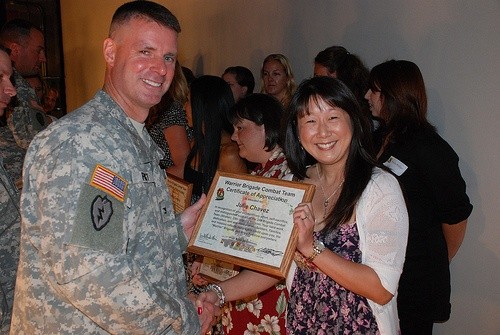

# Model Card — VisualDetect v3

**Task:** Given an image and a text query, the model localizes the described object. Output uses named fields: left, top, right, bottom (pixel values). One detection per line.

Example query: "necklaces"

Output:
left=315, top=163, right=344, bottom=219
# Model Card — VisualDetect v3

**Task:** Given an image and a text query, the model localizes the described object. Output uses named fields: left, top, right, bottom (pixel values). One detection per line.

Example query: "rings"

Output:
left=301, top=213, right=309, bottom=221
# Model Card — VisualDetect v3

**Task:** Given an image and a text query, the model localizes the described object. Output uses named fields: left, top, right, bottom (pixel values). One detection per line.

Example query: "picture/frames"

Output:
left=186, top=170, right=316, bottom=279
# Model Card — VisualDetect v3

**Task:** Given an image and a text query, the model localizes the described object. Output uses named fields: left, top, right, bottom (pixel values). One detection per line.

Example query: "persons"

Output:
left=143, top=45, right=472, bottom=335
left=10, top=0, right=222, bottom=335
left=0, top=19, right=65, bottom=335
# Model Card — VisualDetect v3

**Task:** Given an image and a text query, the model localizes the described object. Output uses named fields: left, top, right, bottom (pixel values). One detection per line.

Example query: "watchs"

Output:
left=306, top=240, right=326, bottom=262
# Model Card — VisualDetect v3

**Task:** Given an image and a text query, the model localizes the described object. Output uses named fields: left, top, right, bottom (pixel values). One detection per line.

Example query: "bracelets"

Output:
left=205, top=283, right=225, bottom=308
